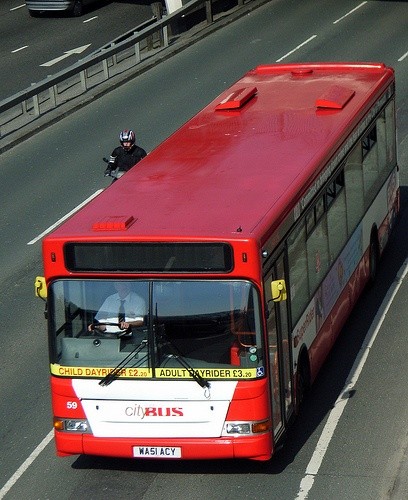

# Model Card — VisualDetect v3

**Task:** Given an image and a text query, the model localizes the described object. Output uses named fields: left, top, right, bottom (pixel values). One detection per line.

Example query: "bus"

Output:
left=33, top=61, right=401, bottom=461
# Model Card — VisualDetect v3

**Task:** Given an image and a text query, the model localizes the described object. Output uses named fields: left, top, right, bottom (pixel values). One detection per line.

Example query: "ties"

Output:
left=118, top=300, right=126, bottom=330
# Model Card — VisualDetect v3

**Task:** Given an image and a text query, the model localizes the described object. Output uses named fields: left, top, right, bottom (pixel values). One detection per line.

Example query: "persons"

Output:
left=88, top=279, right=146, bottom=335
left=104, top=130, right=147, bottom=184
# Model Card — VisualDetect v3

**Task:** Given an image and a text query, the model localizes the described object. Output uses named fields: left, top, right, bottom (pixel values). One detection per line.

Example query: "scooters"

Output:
left=103, top=157, right=133, bottom=184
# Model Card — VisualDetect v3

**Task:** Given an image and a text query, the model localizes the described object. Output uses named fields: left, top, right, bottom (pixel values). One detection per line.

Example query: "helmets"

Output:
left=119, top=129, right=135, bottom=151
